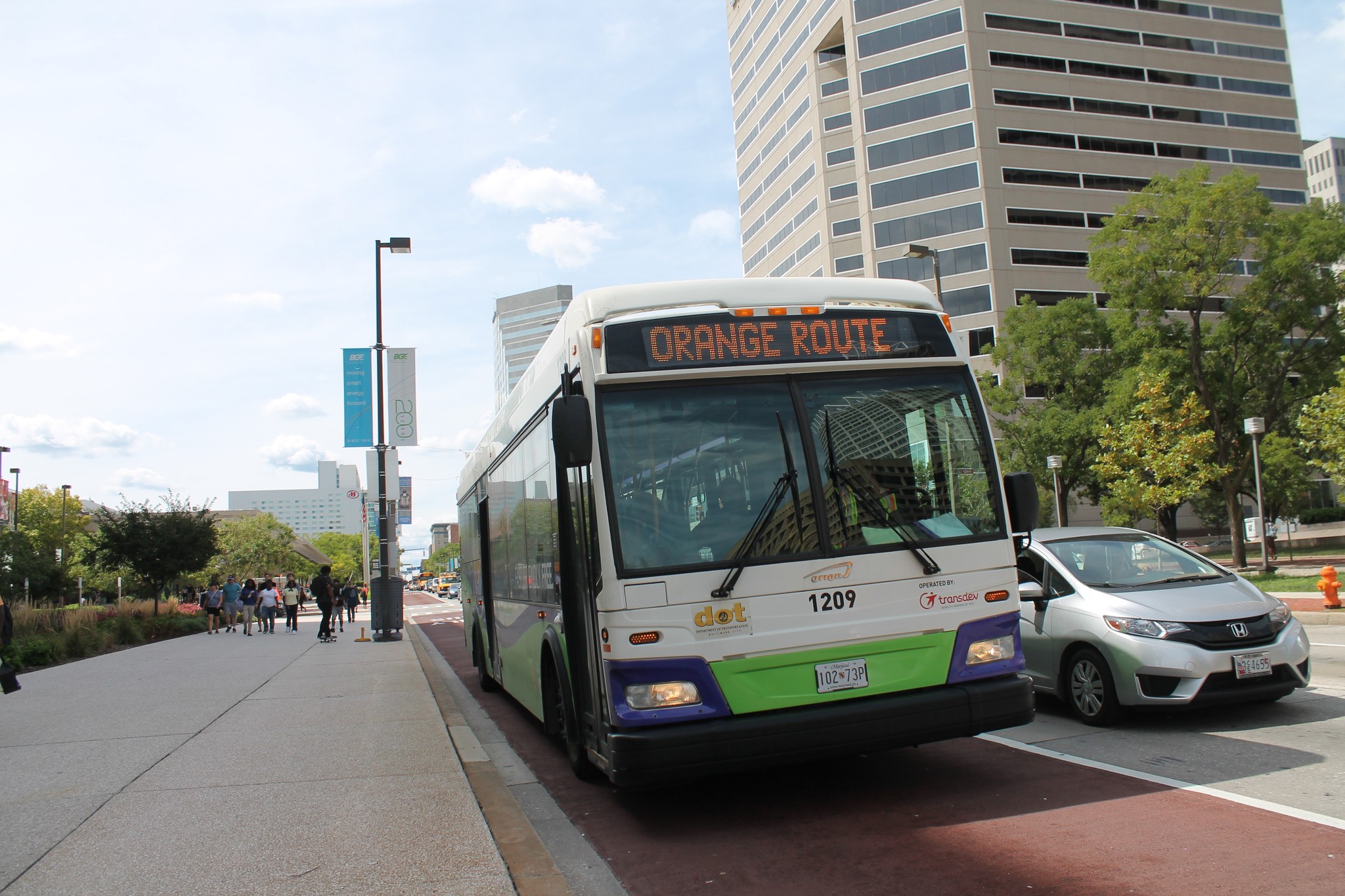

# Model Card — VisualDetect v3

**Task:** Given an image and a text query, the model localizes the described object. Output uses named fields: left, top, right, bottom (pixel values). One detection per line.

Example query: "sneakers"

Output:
left=326, top=635, right=337, bottom=639
left=317, top=635, right=326, bottom=640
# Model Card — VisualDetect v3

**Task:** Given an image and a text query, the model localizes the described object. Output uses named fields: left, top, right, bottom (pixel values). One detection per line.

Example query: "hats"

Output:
left=228, top=575, right=234, bottom=579
left=209, top=583, right=216, bottom=586
left=286, top=571, right=294, bottom=577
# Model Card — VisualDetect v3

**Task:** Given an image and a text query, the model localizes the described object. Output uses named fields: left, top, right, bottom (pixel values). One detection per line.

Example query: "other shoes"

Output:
left=271, top=630, right=274, bottom=633
left=244, top=627, right=247, bottom=635
left=226, top=626, right=231, bottom=633
left=292, top=630, right=296, bottom=634
left=248, top=633, right=252, bottom=636
left=269, top=630, right=271, bottom=632
left=352, top=616, right=355, bottom=623
left=233, top=628, right=236, bottom=632
left=263, top=629, right=269, bottom=633
left=330, top=628, right=335, bottom=632
left=286, top=626, right=290, bottom=632
left=215, top=631, right=219, bottom=634
left=208, top=631, right=212, bottom=634
left=340, top=628, right=344, bottom=632
left=258, top=630, right=262, bottom=632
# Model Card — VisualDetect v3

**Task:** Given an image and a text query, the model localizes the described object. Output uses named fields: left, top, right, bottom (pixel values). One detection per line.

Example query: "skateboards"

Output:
left=320, top=636, right=337, bottom=643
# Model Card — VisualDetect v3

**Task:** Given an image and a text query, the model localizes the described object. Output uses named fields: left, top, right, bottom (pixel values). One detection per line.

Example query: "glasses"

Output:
left=840, top=467, right=854, bottom=472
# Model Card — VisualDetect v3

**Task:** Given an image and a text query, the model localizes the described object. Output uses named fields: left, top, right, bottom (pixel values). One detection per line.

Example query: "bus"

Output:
left=456, top=276, right=1043, bottom=789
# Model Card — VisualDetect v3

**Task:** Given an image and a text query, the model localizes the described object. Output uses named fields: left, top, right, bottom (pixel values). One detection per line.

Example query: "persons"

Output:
left=826, top=468, right=902, bottom=551
left=1098, top=545, right=1149, bottom=582
left=1264, top=517, right=1278, bottom=560
left=692, top=478, right=759, bottom=562
left=202, top=565, right=368, bottom=639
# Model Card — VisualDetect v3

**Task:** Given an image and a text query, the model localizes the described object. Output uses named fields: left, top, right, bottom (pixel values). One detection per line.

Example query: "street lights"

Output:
left=1047, top=455, right=1065, bottom=528
left=10, top=468, right=21, bottom=561
left=1243, top=416, right=1279, bottom=573
left=902, top=244, right=946, bottom=311
left=60, top=485, right=72, bottom=608
left=0, top=446, right=11, bottom=479
left=374, top=237, right=412, bottom=644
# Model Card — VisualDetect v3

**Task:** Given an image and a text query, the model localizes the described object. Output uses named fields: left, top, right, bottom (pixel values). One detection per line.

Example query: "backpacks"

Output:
left=310, top=578, right=323, bottom=595
left=335, top=594, right=344, bottom=606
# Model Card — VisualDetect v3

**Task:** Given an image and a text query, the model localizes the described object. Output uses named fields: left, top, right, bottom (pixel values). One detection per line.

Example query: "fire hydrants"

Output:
left=1315, top=563, right=1343, bottom=609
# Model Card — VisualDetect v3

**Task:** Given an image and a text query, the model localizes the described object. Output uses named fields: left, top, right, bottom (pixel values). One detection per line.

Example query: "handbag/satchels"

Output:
left=204, top=608, right=206, bottom=611
left=360, top=590, right=365, bottom=598
left=235, top=598, right=244, bottom=612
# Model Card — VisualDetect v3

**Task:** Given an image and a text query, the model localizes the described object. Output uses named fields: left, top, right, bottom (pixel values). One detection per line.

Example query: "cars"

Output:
left=403, top=572, right=463, bottom=604
left=1013, top=525, right=1312, bottom=726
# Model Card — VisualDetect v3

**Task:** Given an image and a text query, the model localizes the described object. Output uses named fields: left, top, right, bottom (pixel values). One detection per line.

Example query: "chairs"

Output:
left=1017, top=556, right=1043, bottom=589
left=1081, top=547, right=1110, bottom=581
